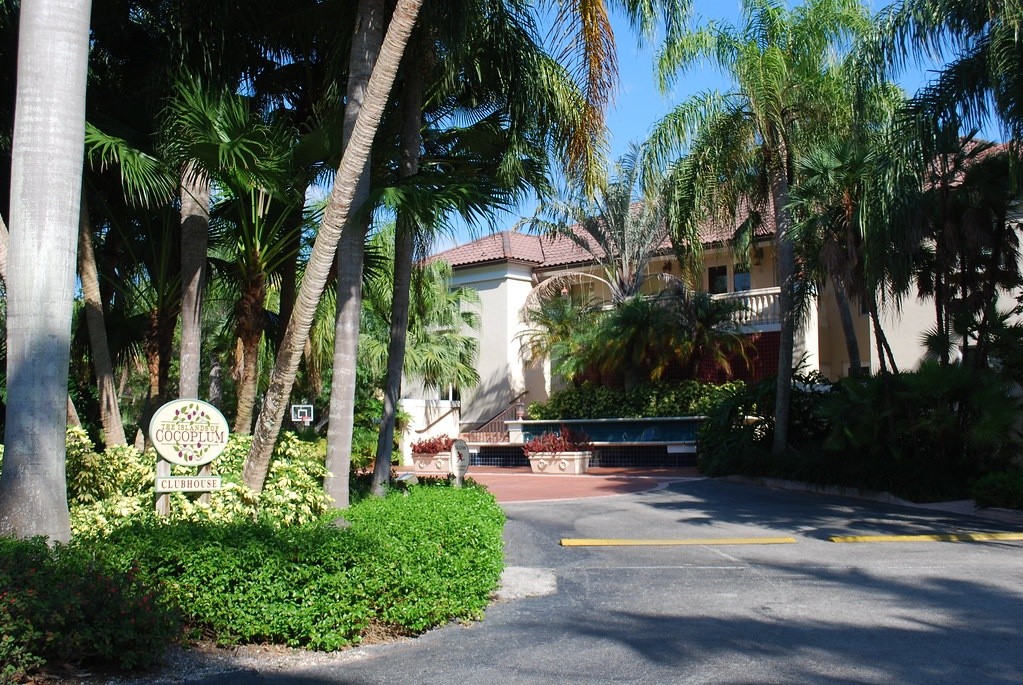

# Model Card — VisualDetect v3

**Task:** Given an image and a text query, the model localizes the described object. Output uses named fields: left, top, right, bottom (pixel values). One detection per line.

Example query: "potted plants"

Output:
left=409, top=433, right=457, bottom=472
left=521, top=421, right=594, bottom=475
